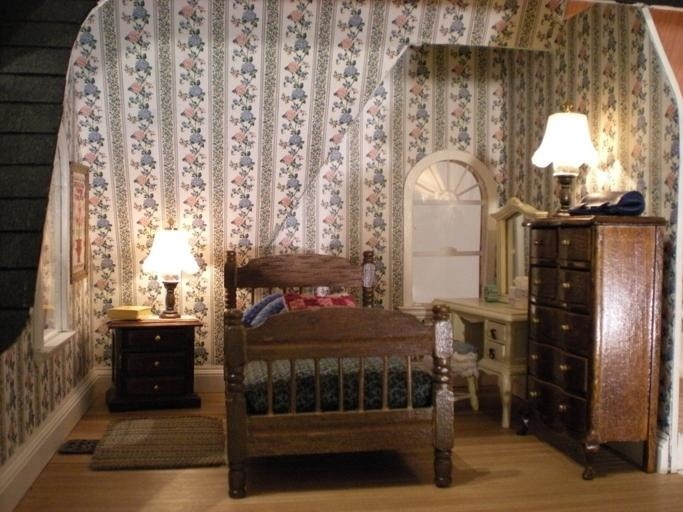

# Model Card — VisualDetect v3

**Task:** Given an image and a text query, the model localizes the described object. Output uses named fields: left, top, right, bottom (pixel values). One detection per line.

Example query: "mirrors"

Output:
left=492, top=198, right=547, bottom=298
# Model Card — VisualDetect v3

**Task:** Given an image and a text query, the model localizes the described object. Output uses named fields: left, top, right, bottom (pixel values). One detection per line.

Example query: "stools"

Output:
left=452, top=340, right=479, bottom=411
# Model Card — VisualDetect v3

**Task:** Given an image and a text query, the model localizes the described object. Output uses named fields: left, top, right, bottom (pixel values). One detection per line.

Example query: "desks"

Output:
left=434, top=298, right=528, bottom=429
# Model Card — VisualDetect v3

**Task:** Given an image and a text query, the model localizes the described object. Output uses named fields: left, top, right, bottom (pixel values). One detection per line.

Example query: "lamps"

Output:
left=142, top=230, right=200, bottom=319
left=532, top=103, right=603, bottom=217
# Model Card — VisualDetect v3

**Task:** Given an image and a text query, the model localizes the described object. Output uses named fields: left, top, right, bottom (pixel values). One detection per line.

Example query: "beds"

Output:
left=224, top=250, right=456, bottom=497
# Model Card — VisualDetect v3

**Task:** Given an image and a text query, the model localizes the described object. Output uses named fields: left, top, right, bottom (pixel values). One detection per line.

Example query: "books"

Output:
left=106, top=303, right=154, bottom=322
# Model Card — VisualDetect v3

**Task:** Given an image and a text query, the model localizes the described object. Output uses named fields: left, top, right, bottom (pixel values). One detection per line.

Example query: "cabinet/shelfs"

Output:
left=516, top=215, right=667, bottom=480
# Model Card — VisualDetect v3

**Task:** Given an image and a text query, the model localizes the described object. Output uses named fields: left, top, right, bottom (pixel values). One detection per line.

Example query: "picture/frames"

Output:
left=70, top=161, right=89, bottom=284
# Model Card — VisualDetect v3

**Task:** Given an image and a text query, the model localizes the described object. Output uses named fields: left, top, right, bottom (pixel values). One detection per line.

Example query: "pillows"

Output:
left=281, top=293, right=358, bottom=309
left=242, top=294, right=283, bottom=327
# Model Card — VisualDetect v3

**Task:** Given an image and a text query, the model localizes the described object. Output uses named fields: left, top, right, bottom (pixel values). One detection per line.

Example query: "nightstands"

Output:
left=105, top=315, right=203, bottom=413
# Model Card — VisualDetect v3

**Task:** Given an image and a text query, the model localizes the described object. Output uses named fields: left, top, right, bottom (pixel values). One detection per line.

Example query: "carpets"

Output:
left=89, top=417, right=226, bottom=468
